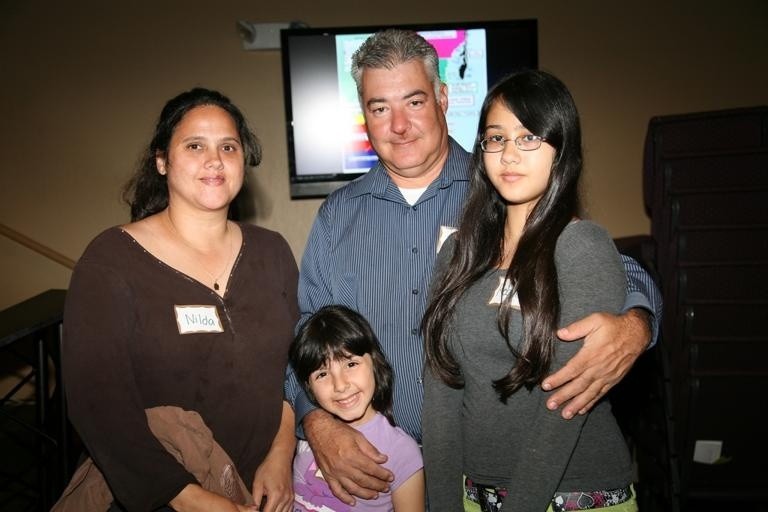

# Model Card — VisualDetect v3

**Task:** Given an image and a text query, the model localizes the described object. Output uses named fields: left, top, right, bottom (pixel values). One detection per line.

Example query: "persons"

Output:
left=422, top=70, right=638, bottom=509
left=284, top=30, right=663, bottom=512
left=54, top=88, right=299, bottom=512
left=290, top=306, right=425, bottom=512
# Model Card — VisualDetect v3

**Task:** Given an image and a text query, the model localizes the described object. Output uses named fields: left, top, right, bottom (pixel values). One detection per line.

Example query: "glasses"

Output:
left=480, top=135, right=544, bottom=152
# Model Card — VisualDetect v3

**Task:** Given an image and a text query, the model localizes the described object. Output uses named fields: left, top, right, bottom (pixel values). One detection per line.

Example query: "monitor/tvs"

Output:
left=277, top=16, right=539, bottom=200
